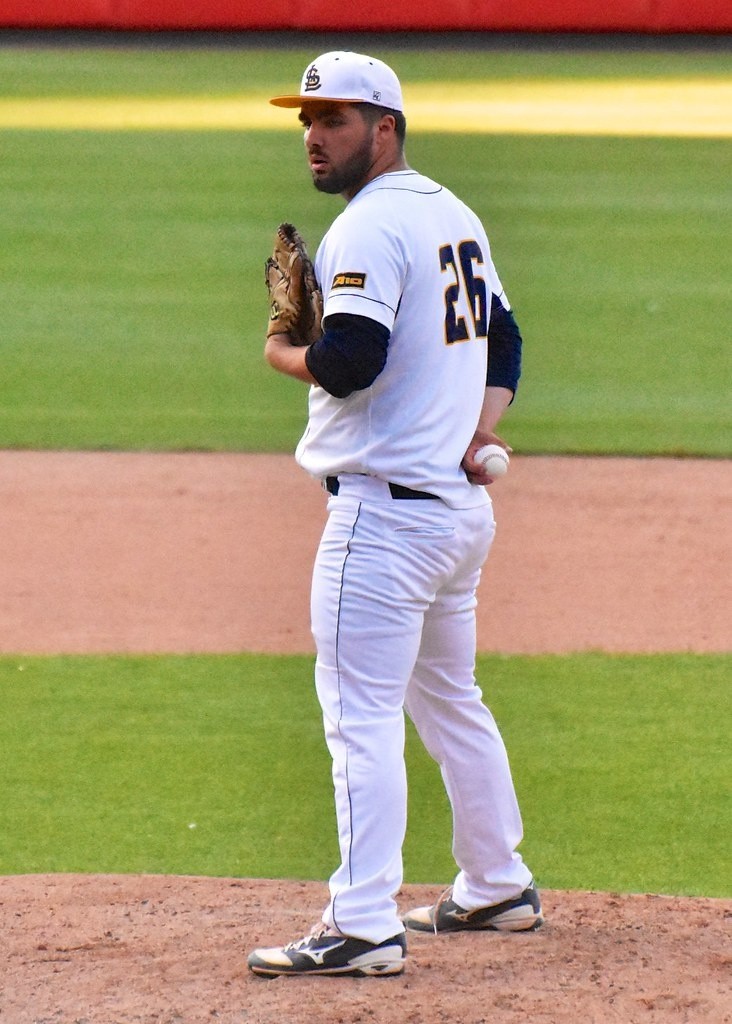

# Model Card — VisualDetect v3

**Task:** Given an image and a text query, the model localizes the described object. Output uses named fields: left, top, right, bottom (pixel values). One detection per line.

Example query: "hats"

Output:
left=268, top=51, right=403, bottom=112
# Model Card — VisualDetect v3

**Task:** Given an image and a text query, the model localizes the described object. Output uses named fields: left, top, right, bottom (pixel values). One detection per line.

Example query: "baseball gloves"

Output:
left=264, top=221, right=325, bottom=347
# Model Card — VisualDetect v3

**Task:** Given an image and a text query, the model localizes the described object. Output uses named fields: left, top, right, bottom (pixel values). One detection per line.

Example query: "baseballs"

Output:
left=473, top=443, right=509, bottom=479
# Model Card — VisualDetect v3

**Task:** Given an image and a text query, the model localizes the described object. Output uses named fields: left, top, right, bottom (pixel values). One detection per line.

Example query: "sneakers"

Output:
left=402, top=878, right=544, bottom=932
left=246, top=920, right=407, bottom=977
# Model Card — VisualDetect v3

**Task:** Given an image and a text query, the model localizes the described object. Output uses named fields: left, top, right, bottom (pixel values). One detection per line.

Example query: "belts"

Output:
left=321, top=476, right=441, bottom=500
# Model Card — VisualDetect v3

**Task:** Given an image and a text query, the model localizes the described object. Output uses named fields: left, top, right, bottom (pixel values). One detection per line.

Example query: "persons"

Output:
left=246, top=51, right=543, bottom=979
